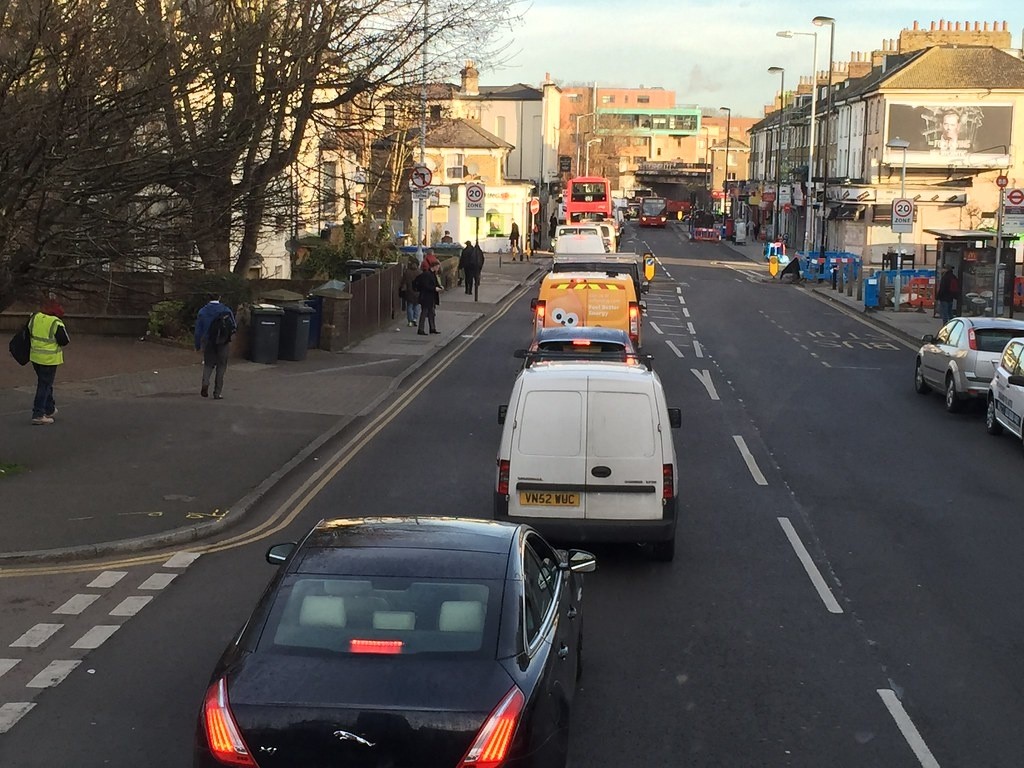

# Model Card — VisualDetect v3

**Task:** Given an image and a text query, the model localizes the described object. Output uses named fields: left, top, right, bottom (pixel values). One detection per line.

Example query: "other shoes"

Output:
left=430, top=331, right=441, bottom=334
left=32, top=415, right=55, bottom=424
left=201, top=383, right=208, bottom=397
left=465, top=291, right=468, bottom=294
left=47, top=408, right=59, bottom=418
left=214, top=395, right=223, bottom=399
left=417, top=330, right=428, bottom=335
left=469, top=292, right=472, bottom=295
left=408, top=321, right=412, bottom=327
left=412, top=318, right=417, bottom=326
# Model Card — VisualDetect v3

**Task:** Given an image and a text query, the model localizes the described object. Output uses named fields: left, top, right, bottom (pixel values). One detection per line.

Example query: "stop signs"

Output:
left=531, top=200, right=539, bottom=215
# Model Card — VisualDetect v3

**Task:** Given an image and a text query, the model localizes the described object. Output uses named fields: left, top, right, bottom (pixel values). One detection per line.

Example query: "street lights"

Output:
left=577, top=111, right=601, bottom=177
left=701, top=107, right=730, bottom=233
left=886, top=137, right=910, bottom=312
left=768, top=66, right=785, bottom=242
left=776, top=16, right=835, bottom=282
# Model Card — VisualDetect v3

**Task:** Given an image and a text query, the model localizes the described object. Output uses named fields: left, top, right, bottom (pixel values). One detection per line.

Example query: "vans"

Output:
left=493, top=350, right=682, bottom=561
left=530, top=269, right=647, bottom=350
left=613, top=200, right=624, bottom=232
left=555, top=218, right=619, bottom=253
left=552, top=234, right=608, bottom=254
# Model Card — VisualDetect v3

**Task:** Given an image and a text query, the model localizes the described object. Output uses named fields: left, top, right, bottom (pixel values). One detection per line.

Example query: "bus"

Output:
left=566, top=177, right=612, bottom=225
left=639, top=196, right=667, bottom=228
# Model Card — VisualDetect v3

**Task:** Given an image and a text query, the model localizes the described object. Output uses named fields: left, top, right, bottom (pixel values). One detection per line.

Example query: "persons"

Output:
left=550, top=213, right=557, bottom=237
left=938, top=264, right=957, bottom=325
left=509, top=218, right=520, bottom=253
left=927, top=110, right=969, bottom=151
left=417, top=261, right=444, bottom=336
left=754, top=223, right=760, bottom=240
left=27, top=299, right=70, bottom=425
left=458, top=241, right=484, bottom=295
left=420, top=249, right=438, bottom=272
left=194, top=288, right=237, bottom=398
left=400, top=258, right=422, bottom=326
left=442, top=231, right=453, bottom=244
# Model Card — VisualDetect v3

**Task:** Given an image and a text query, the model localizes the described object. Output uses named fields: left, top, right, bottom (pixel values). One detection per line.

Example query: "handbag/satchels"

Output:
left=399, top=282, right=407, bottom=297
left=457, top=269, right=462, bottom=285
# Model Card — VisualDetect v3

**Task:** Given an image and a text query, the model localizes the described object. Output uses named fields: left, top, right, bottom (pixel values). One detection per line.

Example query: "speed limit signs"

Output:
left=465, top=183, right=485, bottom=217
left=892, top=198, right=913, bottom=233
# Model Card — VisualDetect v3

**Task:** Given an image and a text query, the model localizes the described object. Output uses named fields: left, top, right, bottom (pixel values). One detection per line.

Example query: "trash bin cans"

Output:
left=282, top=305, right=315, bottom=360
left=725, top=219, right=733, bottom=240
left=434, top=242, right=464, bottom=285
left=250, top=304, right=285, bottom=363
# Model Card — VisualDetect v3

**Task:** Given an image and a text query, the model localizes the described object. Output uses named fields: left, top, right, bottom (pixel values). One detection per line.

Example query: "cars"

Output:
left=913, top=316, right=1024, bottom=444
left=194, top=513, right=597, bottom=768
left=514, top=328, right=652, bottom=372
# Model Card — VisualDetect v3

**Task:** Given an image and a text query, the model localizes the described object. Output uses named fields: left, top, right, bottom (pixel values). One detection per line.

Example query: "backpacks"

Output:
left=9, top=312, right=38, bottom=366
left=210, top=303, right=235, bottom=353
left=412, top=273, right=424, bottom=292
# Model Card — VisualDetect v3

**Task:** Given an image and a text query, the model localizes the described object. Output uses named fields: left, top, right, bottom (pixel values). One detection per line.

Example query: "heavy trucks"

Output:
left=624, top=186, right=692, bottom=221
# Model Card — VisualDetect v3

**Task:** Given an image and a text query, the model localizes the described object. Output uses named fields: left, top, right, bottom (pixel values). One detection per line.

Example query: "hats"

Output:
left=464, top=241, right=472, bottom=245
left=944, top=263, right=953, bottom=271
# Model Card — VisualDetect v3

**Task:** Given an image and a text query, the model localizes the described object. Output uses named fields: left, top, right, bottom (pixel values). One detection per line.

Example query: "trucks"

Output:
left=549, top=252, right=649, bottom=305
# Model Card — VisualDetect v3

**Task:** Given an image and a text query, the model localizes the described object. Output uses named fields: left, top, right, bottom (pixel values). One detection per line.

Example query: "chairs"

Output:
left=323, top=581, right=392, bottom=624
